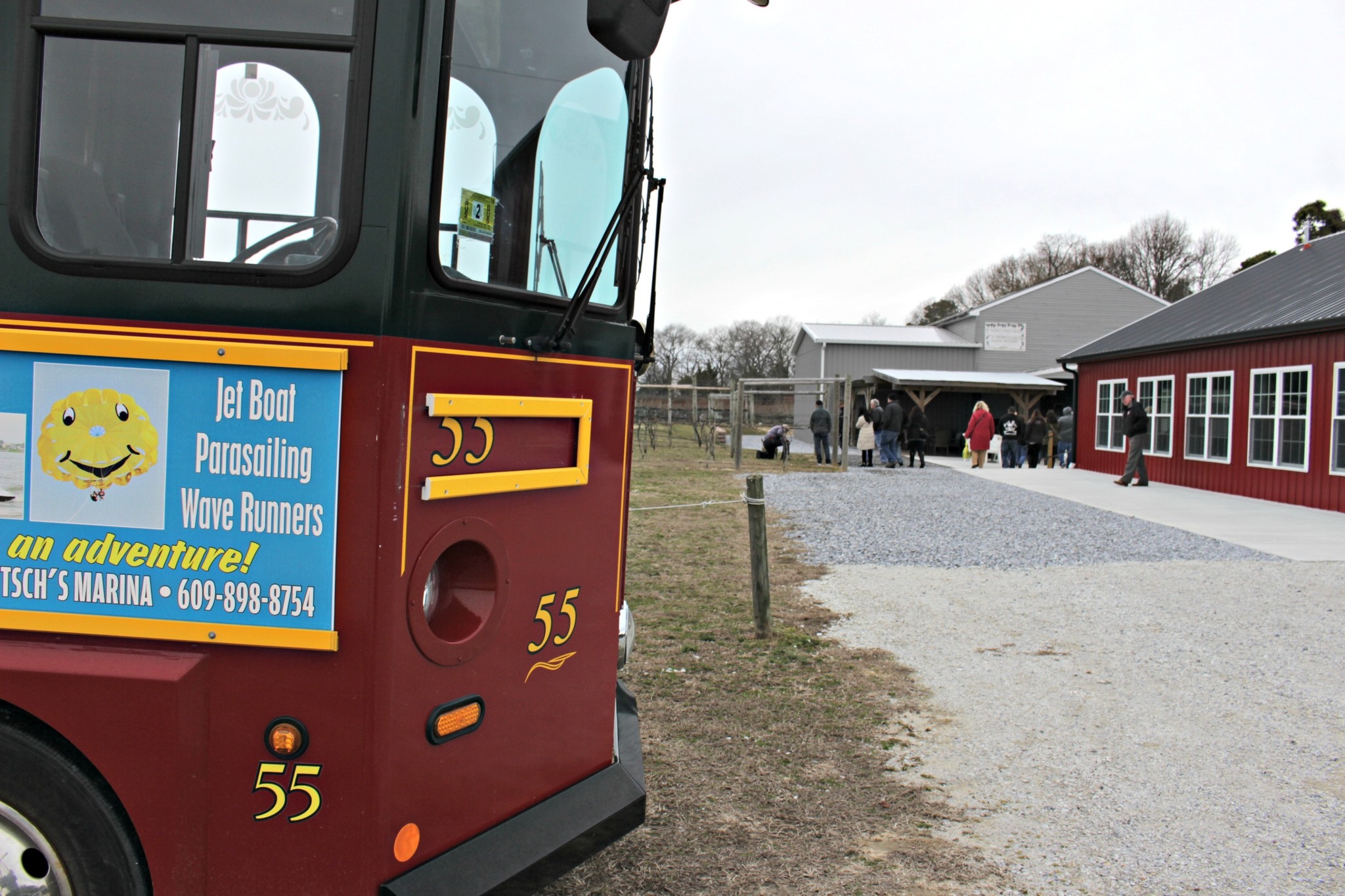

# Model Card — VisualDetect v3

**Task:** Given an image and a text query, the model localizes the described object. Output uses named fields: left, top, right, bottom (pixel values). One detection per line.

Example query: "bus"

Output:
left=0, top=0, right=679, bottom=896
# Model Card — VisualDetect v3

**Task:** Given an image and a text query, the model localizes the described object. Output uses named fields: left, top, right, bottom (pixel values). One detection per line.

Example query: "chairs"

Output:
left=932, top=432, right=961, bottom=455
left=985, top=435, right=1002, bottom=464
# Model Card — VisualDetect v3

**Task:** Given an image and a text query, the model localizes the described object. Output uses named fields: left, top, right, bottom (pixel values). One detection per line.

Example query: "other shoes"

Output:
left=919, top=463, right=924, bottom=468
left=886, top=461, right=898, bottom=468
left=1061, top=463, right=1066, bottom=468
left=1114, top=480, right=1128, bottom=487
left=825, top=463, right=831, bottom=465
left=1133, top=483, right=1147, bottom=486
left=858, top=463, right=873, bottom=467
left=817, top=463, right=821, bottom=465
left=756, top=450, right=761, bottom=458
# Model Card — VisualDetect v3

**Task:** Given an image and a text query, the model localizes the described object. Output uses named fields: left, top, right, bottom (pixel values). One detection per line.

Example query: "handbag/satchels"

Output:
left=920, top=429, right=928, bottom=438
left=963, top=439, right=971, bottom=460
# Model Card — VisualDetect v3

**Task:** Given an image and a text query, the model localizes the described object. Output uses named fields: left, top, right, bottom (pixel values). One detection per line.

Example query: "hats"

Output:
left=1118, top=391, right=1132, bottom=399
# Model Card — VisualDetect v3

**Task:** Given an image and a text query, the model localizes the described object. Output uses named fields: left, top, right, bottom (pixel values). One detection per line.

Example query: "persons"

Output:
left=753, top=394, right=1075, bottom=469
left=1113, top=391, right=1151, bottom=486
